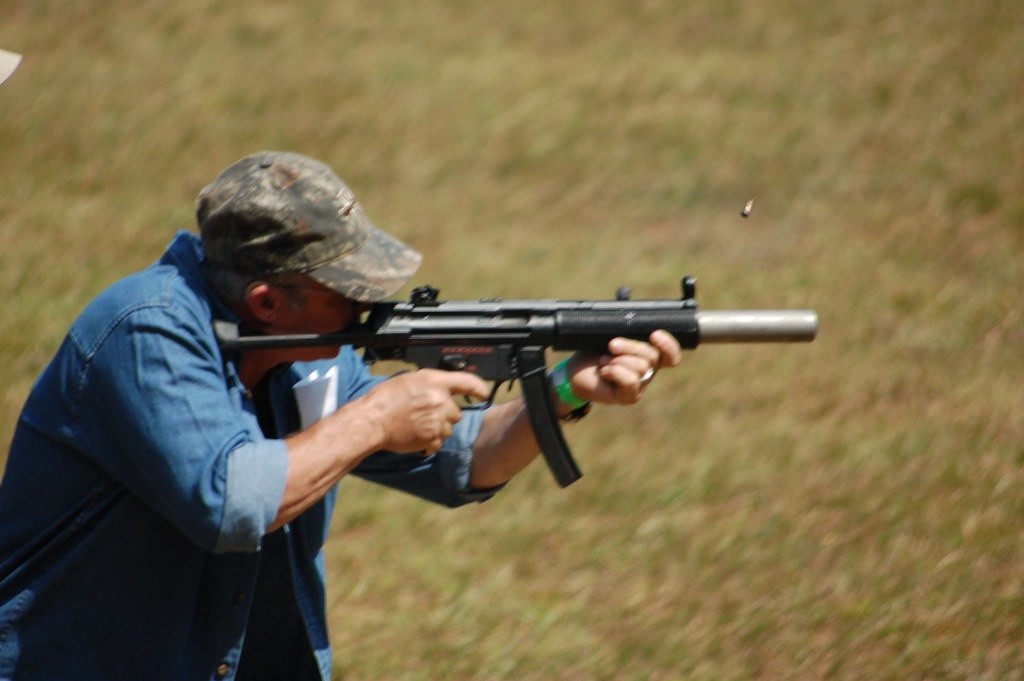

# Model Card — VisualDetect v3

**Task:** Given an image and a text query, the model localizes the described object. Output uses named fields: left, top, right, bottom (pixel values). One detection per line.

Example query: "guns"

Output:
left=212, top=275, right=820, bottom=489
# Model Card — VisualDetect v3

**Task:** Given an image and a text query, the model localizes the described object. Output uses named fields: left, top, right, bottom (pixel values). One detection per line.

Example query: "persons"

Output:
left=0, top=151, right=685, bottom=681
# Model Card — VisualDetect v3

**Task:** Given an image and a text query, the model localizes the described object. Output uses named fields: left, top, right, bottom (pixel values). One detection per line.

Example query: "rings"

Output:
left=641, top=368, right=655, bottom=385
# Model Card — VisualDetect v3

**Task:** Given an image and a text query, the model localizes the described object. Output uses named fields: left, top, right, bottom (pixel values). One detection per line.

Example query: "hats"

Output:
left=193, top=151, right=425, bottom=305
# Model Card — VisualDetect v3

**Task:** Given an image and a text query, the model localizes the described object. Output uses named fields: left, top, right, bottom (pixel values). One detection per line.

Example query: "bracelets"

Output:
left=552, top=357, right=588, bottom=411
